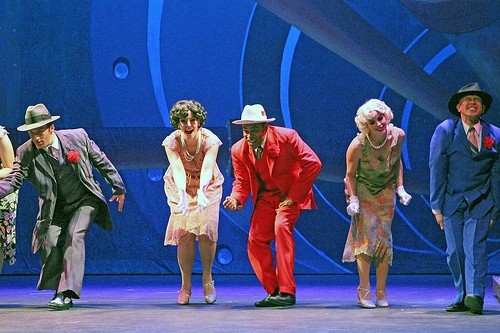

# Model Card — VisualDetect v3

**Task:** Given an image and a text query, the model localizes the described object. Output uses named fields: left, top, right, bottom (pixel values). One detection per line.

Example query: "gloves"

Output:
left=347, top=196, right=360, bottom=215
left=396, top=186, right=412, bottom=206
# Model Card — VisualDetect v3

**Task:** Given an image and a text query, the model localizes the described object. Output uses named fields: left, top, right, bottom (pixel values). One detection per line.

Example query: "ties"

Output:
left=468, top=128, right=478, bottom=149
left=48, top=147, right=59, bottom=160
left=256, top=146, right=261, bottom=160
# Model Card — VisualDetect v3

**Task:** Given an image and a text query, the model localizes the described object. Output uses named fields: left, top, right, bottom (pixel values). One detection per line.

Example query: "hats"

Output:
left=448, top=82, right=493, bottom=117
left=16, top=103, right=61, bottom=132
left=231, top=104, right=275, bottom=124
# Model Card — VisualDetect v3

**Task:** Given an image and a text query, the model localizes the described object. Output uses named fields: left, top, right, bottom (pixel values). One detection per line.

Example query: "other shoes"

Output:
left=202, top=281, right=217, bottom=304
left=177, top=289, right=191, bottom=305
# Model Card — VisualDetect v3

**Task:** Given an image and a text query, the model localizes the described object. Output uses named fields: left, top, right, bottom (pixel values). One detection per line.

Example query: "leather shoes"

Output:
left=446, top=303, right=467, bottom=312
left=269, top=292, right=295, bottom=307
left=255, top=294, right=276, bottom=307
left=47, top=292, right=73, bottom=310
left=465, top=294, right=484, bottom=315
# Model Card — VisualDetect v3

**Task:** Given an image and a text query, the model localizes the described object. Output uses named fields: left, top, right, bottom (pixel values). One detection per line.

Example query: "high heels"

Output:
left=377, top=289, right=389, bottom=306
left=356, top=286, right=376, bottom=308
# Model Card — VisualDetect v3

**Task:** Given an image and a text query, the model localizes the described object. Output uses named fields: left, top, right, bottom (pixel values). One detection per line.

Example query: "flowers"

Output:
left=268, top=144, right=280, bottom=158
left=67, top=150, right=79, bottom=162
left=483, top=136, right=494, bottom=148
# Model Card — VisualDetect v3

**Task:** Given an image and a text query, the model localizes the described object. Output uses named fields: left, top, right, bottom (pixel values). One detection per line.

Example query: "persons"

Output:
left=162, top=99, right=225, bottom=305
left=429, top=82, right=500, bottom=315
left=342, top=98, right=413, bottom=308
left=0, top=104, right=126, bottom=311
left=223, top=103, right=322, bottom=307
left=0, top=125, right=19, bottom=275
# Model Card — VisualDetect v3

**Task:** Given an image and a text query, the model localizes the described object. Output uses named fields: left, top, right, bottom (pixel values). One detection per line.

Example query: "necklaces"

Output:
left=366, top=127, right=388, bottom=149
left=179, top=129, right=201, bottom=161
